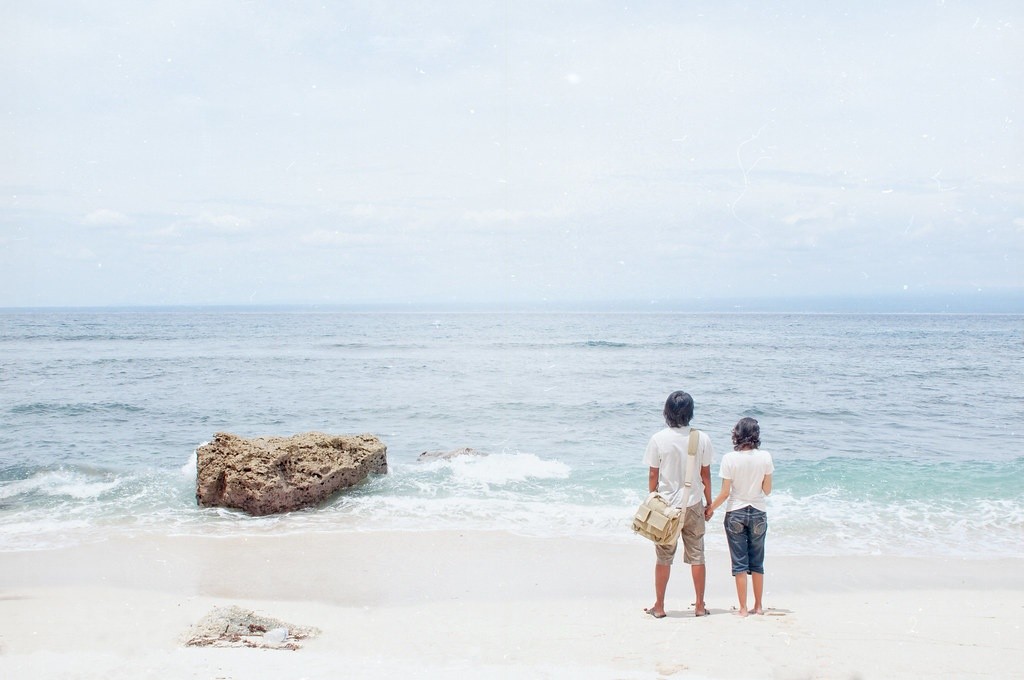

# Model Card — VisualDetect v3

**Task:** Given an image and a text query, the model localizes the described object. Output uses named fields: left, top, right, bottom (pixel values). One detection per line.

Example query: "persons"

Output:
left=706, top=417, right=775, bottom=617
left=646, top=391, right=714, bottom=618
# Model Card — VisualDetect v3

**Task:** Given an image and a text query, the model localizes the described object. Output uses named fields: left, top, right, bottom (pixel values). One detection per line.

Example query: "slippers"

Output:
left=695, top=609, right=710, bottom=617
left=646, top=608, right=666, bottom=619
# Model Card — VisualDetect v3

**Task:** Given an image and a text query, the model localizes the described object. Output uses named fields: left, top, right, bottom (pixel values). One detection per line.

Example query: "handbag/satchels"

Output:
left=631, top=490, right=685, bottom=545
